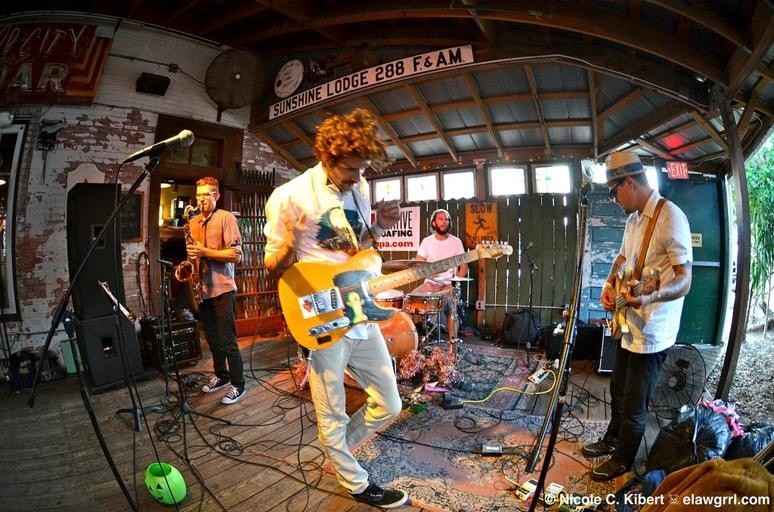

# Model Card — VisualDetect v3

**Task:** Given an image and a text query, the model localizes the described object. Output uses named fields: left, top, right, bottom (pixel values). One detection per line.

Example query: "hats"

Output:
left=606, top=152, right=645, bottom=180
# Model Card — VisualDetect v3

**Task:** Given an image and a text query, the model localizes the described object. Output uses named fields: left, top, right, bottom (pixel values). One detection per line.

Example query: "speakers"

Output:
left=66, top=183, right=145, bottom=394
left=136, top=72, right=170, bottom=96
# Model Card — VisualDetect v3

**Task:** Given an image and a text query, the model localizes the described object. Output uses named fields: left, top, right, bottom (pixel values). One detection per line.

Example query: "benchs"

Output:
left=573, top=322, right=604, bottom=360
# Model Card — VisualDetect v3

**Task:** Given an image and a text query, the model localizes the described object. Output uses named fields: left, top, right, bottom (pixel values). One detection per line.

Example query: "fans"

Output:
left=206, top=46, right=269, bottom=122
left=649, top=340, right=706, bottom=431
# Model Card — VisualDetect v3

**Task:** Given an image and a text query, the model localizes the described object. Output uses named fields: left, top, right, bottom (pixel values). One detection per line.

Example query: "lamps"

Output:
left=0, top=107, right=16, bottom=128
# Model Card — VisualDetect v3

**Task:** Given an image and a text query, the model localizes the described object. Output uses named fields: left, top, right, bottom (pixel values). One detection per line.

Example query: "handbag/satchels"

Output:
left=544, top=317, right=585, bottom=358
left=503, top=308, right=540, bottom=346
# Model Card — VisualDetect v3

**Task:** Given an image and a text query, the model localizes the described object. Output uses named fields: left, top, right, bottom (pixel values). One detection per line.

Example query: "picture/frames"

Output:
left=120, top=191, right=144, bottom=243
left=465, top=202, right=500, bottom=251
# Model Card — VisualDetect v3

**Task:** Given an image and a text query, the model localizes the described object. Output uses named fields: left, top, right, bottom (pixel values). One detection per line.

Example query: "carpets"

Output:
left=294, top=341, right=547, bottom=413
left=318, top=400, right=650, bottom=512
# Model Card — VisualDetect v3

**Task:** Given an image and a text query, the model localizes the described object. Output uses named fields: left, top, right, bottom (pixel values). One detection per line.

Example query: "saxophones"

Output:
left=174, top=204, right=205, bottom=314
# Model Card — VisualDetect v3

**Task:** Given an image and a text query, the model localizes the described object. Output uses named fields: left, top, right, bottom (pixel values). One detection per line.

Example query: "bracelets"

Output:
left=371, top=222, right=385, bottom=234
left=601, top=282, right=613, bottom=290
left=274, top=252, right=295, bottom=270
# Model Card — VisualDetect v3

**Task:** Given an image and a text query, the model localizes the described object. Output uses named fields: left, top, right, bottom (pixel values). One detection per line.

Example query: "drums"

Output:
left=405, top=292, right=447, bottom=315
left=344, top=307, right=419, bottom=381
left=375, top=289, right=404, bottom=309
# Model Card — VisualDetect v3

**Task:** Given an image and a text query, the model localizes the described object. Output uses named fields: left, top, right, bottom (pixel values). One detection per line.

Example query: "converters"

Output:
left=481, top=443, right=502, bottom=456
left=443, top=393, right=452, bottom=402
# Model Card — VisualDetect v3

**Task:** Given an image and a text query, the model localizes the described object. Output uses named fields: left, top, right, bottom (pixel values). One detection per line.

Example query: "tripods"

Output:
left=157, top=286, right=231, bottom=459
left=116, top=318, right=161, bottom=432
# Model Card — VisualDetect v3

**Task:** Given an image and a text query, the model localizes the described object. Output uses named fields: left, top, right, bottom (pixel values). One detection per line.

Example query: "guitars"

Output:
left=611, top=268, right=660, bottom=341
left=277, top=240, right=513, bottom=349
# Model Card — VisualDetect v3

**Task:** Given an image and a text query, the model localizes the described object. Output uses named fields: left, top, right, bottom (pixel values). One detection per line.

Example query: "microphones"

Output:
left=524, top=241, right=533, bottom=252
left=123, top=129, right=195, bottom=164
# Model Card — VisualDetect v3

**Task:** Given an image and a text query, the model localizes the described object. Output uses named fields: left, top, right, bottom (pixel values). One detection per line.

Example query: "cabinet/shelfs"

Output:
left=224, top=184, right=283, bottom=338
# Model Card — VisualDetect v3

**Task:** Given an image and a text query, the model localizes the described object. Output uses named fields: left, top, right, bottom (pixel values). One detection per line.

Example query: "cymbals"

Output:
left=381, top=257, right=431, bottom=272
left=449, top=277, right=474, bottom=281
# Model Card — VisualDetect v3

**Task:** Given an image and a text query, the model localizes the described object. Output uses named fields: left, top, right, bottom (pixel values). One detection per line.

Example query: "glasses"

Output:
left=609, top=176, right=634, bottom=199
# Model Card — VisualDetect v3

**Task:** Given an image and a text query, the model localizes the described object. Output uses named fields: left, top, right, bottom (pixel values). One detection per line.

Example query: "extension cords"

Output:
left=528, top=367, right=551, bottom=385
left=442, top=398, right=463, bottom=409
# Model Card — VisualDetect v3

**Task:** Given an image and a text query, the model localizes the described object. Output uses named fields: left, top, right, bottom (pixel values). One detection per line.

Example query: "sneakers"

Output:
left=590, top=459, right=631, bottom=479
left=350, top=482, right=407, bottom=508
left=221, top=386, right=245, bottom=404
left=202, top=376, right=230, bottom=393
left=581, top=439, right=618, bottom=455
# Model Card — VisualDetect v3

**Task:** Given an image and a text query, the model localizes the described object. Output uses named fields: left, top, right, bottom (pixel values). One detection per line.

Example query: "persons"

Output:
left=263, top=109, right=407, bottom=510
left=186, top=177, right=246, bottom=405
left=582, top=151, right=694, bottom=481
left=416, top=209, right=470, bottom=360
